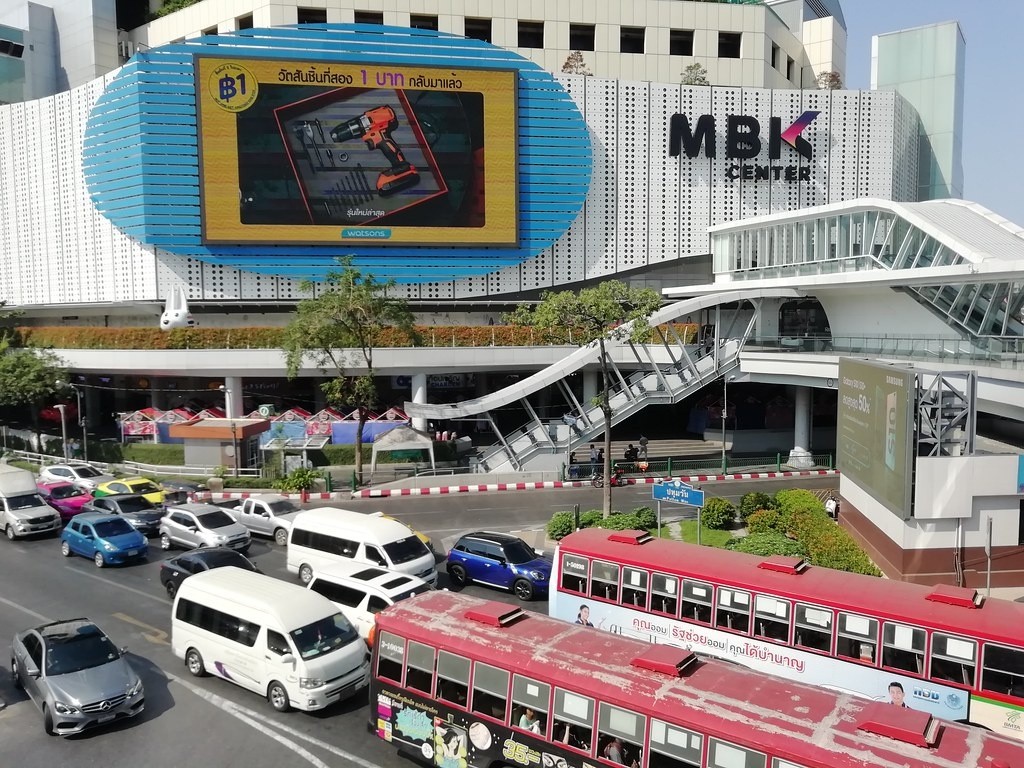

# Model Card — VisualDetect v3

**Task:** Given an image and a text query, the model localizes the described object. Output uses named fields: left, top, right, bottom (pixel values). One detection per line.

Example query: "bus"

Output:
left=365, top=587, right=1024, bottom=768
left=548, top=527, right=1024, bottom=746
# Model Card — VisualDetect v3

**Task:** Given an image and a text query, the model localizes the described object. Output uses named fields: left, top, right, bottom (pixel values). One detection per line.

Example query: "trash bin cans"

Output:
left=570, top=466, right=580, bottom=477
left=780, top=338, right=803, bottom=352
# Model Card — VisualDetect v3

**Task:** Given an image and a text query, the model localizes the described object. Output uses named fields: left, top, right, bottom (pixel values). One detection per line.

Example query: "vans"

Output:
left=286, top=506, right=439, bottom=588
left=0, top=462, right=63, bottom=541
left=169, top=563, right=372, bottom=713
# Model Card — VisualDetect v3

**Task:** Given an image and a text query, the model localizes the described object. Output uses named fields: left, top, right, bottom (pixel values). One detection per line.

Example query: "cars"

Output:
left=36, top=479, right=96, bottom=522
left=80, top=493, right=166, bottom=539
left=59, top=512, right=149, bottom=568
left=94, top=476, right=177, bottom=506
left=157, top=502, right=253, bottom=554
left=159, top=477, right=206, bottom=502
left=159, top=546, right=266, bottom=599
left=447, top=530, right=554, bottom=601
left=366, top=511, right=434, bottom=553
left=9, top=616, right=146, bottom=736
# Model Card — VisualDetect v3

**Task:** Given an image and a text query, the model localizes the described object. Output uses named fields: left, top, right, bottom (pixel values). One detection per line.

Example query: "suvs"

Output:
left=36, top=461, right=118, bottom=494
left=305, top=559, right=438, bottom=654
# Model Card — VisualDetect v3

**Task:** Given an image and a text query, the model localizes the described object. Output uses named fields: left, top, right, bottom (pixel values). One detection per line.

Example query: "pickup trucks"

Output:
left=190, top=493, right=309, bottom=547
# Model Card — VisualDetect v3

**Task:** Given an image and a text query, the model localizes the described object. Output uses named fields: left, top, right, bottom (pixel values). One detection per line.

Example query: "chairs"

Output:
left=579, top=578, right=971, bottom=687
left=407, top=667, right=616, bottom=759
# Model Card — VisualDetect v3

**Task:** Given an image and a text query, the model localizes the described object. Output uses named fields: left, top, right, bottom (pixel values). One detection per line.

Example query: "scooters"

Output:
left=592, top=465, right=625, bottom=489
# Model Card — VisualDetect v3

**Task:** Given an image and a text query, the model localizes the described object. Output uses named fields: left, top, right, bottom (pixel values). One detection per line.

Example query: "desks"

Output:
left=395, top=467, right=415, bottom=480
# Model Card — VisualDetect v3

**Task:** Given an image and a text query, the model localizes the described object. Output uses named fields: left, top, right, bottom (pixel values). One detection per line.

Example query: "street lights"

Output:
left=53, top=403, right=68, bottom=463
left=54, top=379, right=88, bottom=462
left=220, top=385, right=233, bottom=426
left=721, top=375, right=736, bottom=458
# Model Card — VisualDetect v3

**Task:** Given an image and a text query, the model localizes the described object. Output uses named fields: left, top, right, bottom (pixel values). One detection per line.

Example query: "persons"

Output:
left=627, top=433, right=648, bottom=462
left=589, top=444, right=604, bottom=477
left=610, top=737, right=638, bottom=768
left=575, top=604, right=593, bottom=626
left=888, top=682, right=912, bottom=709
left=570, top=452, right=576, bottom=464
left=559, top=722, right=588, bottom=749
left=519, top=706, right=540, bottom=730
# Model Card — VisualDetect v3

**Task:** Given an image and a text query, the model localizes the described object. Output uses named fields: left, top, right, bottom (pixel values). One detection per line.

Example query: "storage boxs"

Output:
left=273, top=87, right=448, bottom=226
